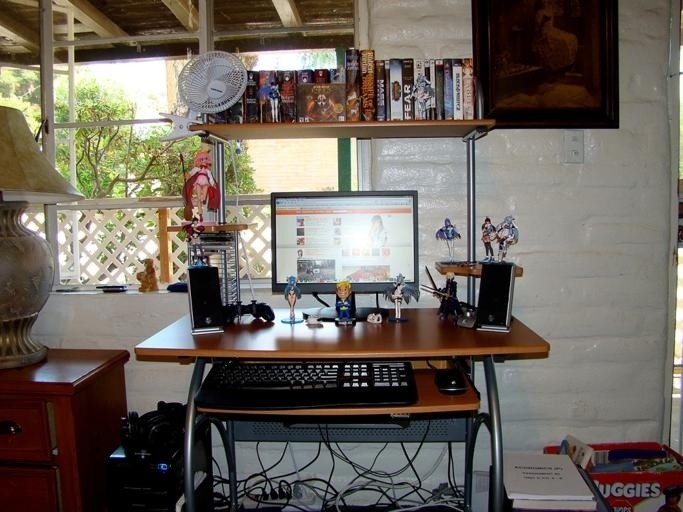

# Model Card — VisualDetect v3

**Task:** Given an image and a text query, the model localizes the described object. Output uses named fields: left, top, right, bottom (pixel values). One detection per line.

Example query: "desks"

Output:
left=133, top=307, right=551, bottom=512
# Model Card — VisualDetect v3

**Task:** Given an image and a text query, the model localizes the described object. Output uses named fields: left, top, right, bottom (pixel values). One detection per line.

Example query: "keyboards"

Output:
left=196, top=360, right=419, bottom=407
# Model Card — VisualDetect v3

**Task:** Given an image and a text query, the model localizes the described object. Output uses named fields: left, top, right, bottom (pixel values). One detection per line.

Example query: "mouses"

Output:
left=434, top=368, right=467, bottom=395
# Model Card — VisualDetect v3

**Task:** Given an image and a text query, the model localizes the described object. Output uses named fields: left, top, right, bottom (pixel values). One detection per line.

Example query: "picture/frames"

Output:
left=471, top=0, right=619, bottom=129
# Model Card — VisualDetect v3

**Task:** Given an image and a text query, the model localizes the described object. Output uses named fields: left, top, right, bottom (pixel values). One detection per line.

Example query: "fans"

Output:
left=160, top=51, right=248, bottom=141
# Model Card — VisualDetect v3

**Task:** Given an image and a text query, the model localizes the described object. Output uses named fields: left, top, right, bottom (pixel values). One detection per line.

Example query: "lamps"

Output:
left=2, top=105, right=85, bottom=372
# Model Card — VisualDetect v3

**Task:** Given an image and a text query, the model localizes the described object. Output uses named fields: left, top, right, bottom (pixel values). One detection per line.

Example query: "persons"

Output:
left=185, top=217, right=206, bottom=264
left=336, top=281, right=352, bottom=319
left=186, top=151, right=216, bottom=222
left=657, top=494, right=681, bottom=512
left=481, top=218, right=497, bottom=262
left=284, top=274, right=302, bottom=319
left=496, top=215, right=520, bottom=263
left=438, top=271, right=463, bottom=320
left=391, top=273, right=405, bottom=318
left=436, top=218, right=461, bottom=262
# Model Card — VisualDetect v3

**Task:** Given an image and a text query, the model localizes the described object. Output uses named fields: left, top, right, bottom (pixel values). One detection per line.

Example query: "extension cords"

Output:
left=243, top=491, right=315, bottom=509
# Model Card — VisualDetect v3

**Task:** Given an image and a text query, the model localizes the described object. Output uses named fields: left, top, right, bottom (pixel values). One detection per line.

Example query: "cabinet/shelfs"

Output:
left=1, top=348, right=129, bottom=510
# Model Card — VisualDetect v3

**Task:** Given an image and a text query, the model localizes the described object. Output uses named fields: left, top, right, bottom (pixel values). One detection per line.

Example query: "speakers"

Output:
left=187, top=266, right=224, bottom=336
left=476, top=262, right=515, bottom=333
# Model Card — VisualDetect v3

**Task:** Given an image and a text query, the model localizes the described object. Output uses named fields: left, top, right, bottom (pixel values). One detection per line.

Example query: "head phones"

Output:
left=121, top=401, right=185, bottom=453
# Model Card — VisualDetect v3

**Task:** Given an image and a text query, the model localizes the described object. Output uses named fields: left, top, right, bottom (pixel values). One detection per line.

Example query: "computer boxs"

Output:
left=105, top=422, right=215, bottom=512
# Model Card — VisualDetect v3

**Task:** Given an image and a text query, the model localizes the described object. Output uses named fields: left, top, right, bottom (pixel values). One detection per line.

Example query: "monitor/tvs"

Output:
left=271, top=189, right=419, bottom=321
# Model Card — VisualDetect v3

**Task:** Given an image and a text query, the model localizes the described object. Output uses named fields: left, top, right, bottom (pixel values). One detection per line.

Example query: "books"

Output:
left=503, top=433, right=597, bottom=512
left=215, top=48, right=476, bottom=124
